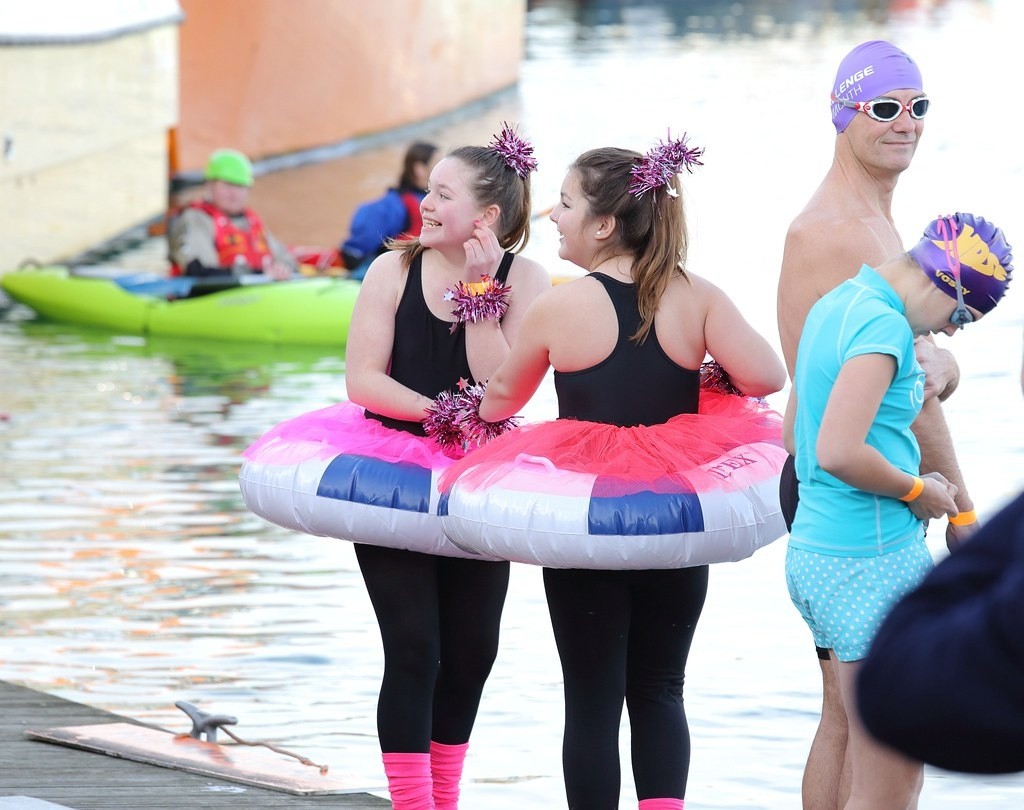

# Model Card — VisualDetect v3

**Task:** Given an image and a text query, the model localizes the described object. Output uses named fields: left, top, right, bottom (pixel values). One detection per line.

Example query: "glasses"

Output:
left=936, top=214, right=976, bottom=331
left=829, top=87, right=932, bottom=123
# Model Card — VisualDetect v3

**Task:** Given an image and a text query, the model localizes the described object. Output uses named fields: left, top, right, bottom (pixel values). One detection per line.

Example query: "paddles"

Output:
left=115, top=268, right=278, bottom=299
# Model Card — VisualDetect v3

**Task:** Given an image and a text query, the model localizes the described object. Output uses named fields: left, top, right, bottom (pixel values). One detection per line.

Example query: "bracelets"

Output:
left=899, top=474, right=925, bottom=503
left=947, top=507, right=978, bottom=527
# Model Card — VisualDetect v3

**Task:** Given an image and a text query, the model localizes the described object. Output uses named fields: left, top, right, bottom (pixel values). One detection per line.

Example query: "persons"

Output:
left=461, top=146, right=788, bottom=809
left=777, top=39, right=982, bottom=809
left=168, top=146, right=303, bottom=283
left=784, top=210, right=1016, bottom=809
left=344, top=144, right=554, bottom=809
left=337, top=139, right=446, bottom=282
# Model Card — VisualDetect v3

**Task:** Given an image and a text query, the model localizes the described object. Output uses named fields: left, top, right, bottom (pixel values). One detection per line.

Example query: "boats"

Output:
left=1, top=266, right=363, bottom=350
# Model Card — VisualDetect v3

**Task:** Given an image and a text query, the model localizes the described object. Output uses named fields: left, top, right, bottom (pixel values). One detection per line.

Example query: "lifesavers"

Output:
left=236, top=435, right=504, bottom=564
left=436, top=443, right=794, bottom=573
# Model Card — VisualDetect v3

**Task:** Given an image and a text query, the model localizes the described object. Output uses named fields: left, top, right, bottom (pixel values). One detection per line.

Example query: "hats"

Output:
left=909, top=212, right=1014, bottom=316
left=206, top=146, right=251, bottom=189
left=831, top=38, right=922, bottom=137
